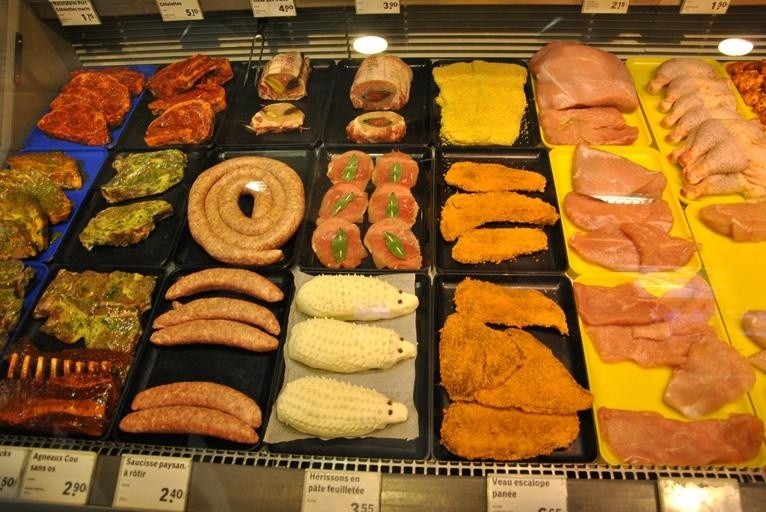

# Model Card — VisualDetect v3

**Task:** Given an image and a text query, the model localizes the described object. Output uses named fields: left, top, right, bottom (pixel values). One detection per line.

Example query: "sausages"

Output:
left=149, top=266, right=285, bottom=352
left=118, top=380, right=263, bottom=445
